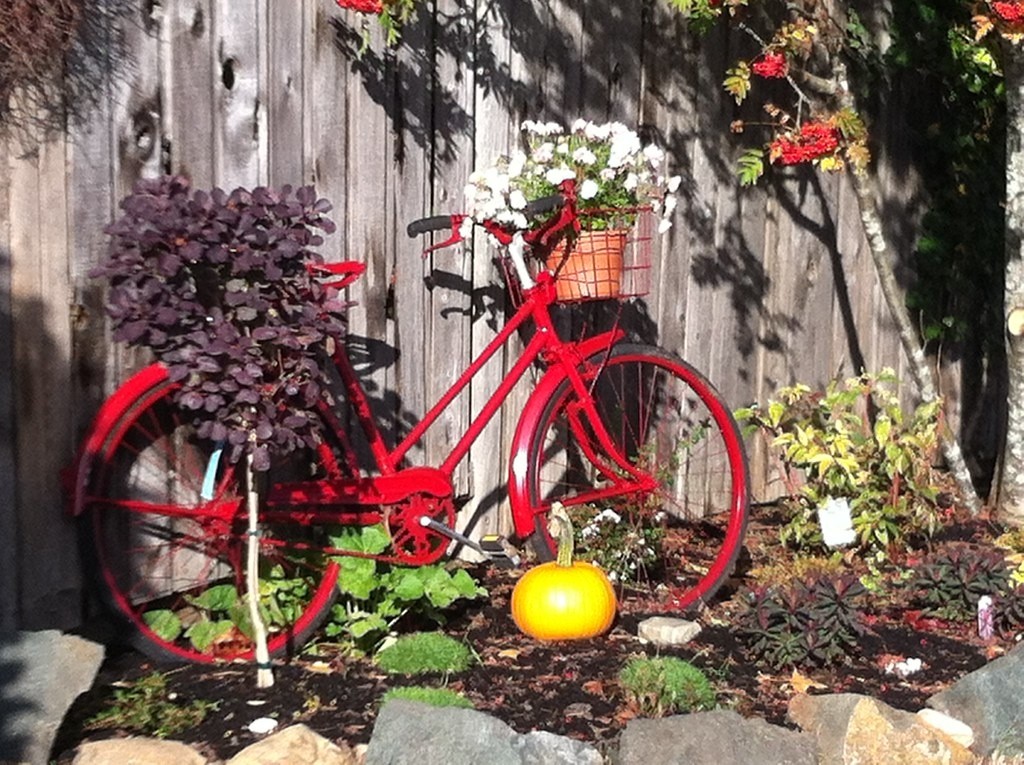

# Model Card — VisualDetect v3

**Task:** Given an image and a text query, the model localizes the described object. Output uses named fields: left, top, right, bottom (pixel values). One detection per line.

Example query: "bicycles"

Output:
left=58, top=178, right=753, bottom=680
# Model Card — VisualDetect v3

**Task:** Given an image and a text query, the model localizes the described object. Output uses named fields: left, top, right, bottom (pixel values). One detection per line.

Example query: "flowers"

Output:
left=458, top=118, right=682, bottom=249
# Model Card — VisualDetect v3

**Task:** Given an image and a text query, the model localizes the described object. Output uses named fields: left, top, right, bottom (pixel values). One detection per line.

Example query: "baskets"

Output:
left=496, top=205, right=652, bottom=309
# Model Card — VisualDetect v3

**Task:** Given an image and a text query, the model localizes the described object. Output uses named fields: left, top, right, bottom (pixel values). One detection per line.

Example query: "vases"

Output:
left=527, top=230, right=629, bottom=299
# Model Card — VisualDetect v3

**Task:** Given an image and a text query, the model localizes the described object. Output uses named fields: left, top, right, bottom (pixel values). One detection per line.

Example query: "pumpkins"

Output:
left=511, top=501, right=617, bottom=640
